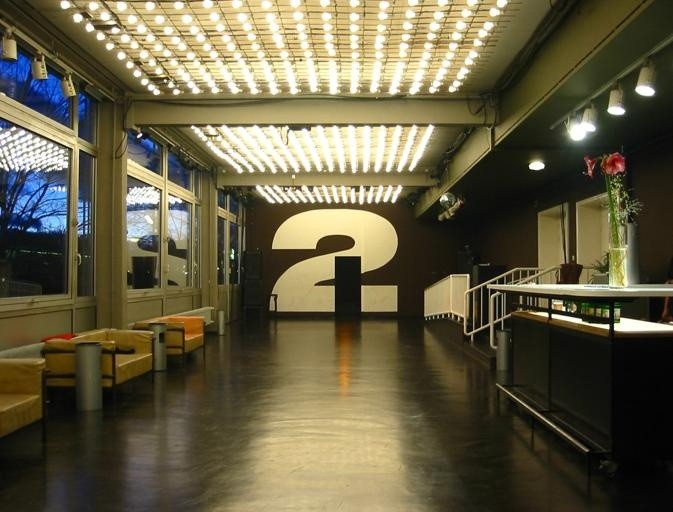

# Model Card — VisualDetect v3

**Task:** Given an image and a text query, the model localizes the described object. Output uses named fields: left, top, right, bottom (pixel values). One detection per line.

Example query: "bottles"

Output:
left=565, top=300, right=621, bottom=323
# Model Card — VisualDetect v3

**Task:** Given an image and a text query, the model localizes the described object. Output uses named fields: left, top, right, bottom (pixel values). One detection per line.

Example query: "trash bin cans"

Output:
left=76, top=342, right=103, bottom=412
left=495, top=329, right=513, bottom=371
left=217, top=310, right=226, bottom=336
left=149, top=323, right=168, bottom=371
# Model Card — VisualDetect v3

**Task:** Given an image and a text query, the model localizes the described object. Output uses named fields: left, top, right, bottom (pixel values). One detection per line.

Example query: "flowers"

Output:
left=582, top=153, right=643, bottom=247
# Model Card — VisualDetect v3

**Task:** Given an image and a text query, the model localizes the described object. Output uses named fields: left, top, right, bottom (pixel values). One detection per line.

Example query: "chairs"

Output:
left=0, top=314, right=205, bottom=441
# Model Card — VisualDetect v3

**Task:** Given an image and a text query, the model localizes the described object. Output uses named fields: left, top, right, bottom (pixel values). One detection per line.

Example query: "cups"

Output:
left=553, top=302, right=563, bottom=311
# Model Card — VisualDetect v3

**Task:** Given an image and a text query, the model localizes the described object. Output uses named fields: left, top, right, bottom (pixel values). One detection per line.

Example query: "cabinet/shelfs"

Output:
left=488, top=283, right=673, bottom=491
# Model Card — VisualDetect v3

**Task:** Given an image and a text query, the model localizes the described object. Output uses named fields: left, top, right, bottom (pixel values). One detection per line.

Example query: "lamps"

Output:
left=567, top=59, right=662, bottom=142
left=1, top=30, right=78, bottom=101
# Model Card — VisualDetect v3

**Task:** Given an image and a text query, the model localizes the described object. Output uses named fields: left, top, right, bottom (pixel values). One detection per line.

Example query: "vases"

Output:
left=608, top=246, right=628, bottom=290
